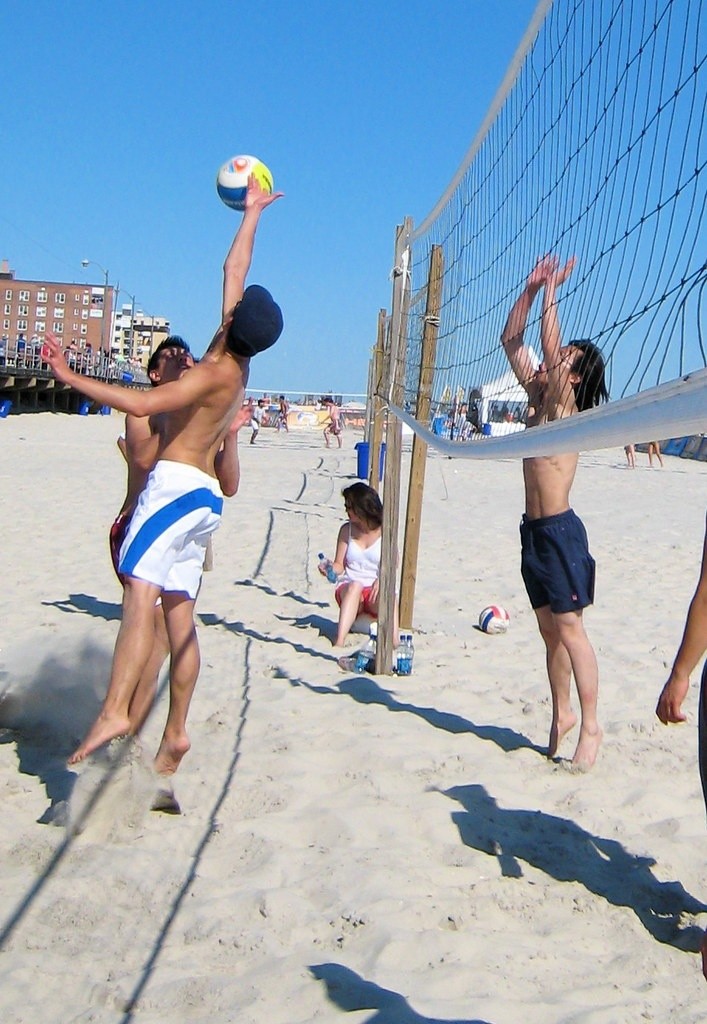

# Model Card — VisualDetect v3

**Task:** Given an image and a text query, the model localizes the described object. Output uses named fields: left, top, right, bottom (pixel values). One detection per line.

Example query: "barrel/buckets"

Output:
left=102, top=404, right=111, bottom=415
left=483, top=423, right=492, bottom=434
left=79, top=401, right=91, bottom=414
left=0, top=401, right=13, bottom=418
left=354, top=442, right=387, bottom=480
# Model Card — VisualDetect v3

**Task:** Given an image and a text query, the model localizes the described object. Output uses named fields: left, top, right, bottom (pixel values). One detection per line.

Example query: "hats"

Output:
left=226, top=284, right=283, bottom=357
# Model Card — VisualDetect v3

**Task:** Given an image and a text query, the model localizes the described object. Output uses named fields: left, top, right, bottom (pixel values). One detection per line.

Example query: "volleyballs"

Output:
left=479, top=606, right=511, bottom=634
left=216, top=154, right=275, bottom=212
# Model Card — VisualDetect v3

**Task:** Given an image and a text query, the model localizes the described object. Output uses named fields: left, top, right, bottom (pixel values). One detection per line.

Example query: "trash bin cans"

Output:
left=483, top=423, right=491, bottom=435
left=353, top=442, right=386, bottom=480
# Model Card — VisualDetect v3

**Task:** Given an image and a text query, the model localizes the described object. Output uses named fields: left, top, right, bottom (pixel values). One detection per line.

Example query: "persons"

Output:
left=647, top=440, right=665, bottom=469
left=39, top=175, right=285, bottom=775
left=657, top=516, right=707, bottom=979
left=319, top=396, right=343, bottom=449
left=275, top=395, right=289, bottom=432
left=316, top=480, right=384, bottom=653
left=498, top=256, right=609, bottom=772
left=249, top=400, right=268, bottom=445
left=0, top=334, right=146, bottom=375
left=625, top=442, right=635, bottom=470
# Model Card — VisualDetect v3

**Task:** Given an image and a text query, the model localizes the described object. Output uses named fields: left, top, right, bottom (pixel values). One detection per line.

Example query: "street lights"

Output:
left=82, top=260, right=108, bottom=376
left=115, top=289, right=135, bottom=361
left=137, top=309, right=154, bottom=357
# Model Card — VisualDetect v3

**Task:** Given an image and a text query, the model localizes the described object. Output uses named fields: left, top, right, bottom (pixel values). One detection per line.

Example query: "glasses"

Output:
left=344, top=504, right=353, bottom=510
left=232, top=300, right=241, bottom=316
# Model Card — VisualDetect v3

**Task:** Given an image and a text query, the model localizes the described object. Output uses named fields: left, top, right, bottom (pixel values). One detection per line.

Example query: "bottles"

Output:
left=396, top=635, right=414, bottom=676
left=318, top=553, right=338, bottom=584
left=353, top=634, right=377, bottom=674
